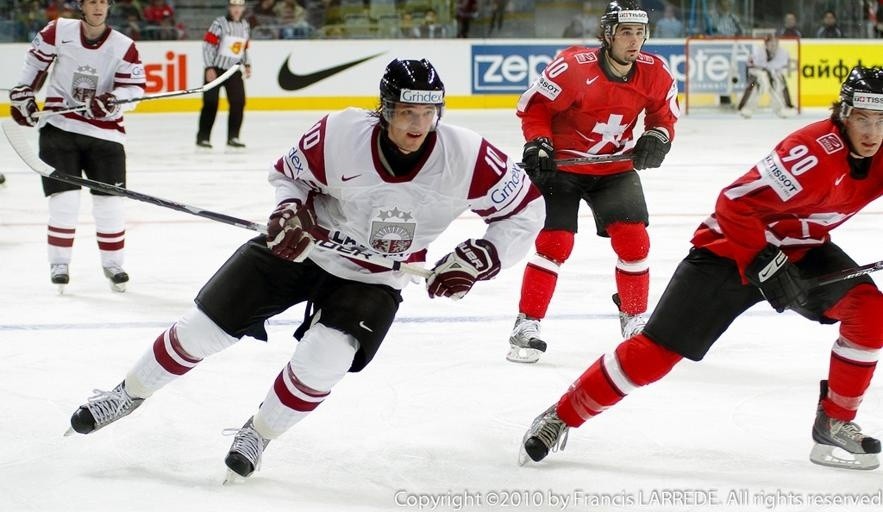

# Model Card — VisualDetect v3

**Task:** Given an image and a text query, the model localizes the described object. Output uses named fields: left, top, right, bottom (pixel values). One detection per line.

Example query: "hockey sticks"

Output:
left=32, top=63, right=240, bottom=121
left=3, top=119, right=433, bottom=278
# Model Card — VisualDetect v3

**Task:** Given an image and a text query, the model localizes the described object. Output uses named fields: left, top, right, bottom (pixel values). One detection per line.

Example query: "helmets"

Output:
left=841, top=66, right=883, bottom=133
left=75, top=0, right=114, bottom=7
left=765, top=32, right=777, bottom=48
left=600, top=0, right=650, bottom=45
left=380, top=59, right=445, bottom=133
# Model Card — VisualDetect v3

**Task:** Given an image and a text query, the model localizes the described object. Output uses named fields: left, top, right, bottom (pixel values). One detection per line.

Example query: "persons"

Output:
left=1, top=1, right=187, bottom=43
left=9, top=2, right=147, bottom=294
left=509, top=2, right=680, bottom=362
left=196, top=1, right=250, bottom=149
left=251, top=0, right=506, bottom=42
left=652, top=7, right=686, bottom=37
left=739, top=30, right=799, bottom=121
left=520, top=64, right=877, bottom=468
left=813, top=12, right=843, bottom=38
left=777, top=13, right=805, bottom=38
left=708, top=0, right=744, bottom=37
left=71, top=58, right=548, bottom=478
left=562, top=2, right=601, bottom=38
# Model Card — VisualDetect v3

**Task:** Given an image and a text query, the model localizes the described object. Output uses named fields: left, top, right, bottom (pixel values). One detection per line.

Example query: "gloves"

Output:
left=746, top=244, right=808, bottom=312
left=267, top=200, right=314, bottom=262
left=631, top=128, right=671, bottom=169
left=425, top=240, right=501, bottom=299
left=86, top=93, right=117, bottom=119
left=523, top=140, right=558, bottom=185
left=10, top=85, right=39, bottom=128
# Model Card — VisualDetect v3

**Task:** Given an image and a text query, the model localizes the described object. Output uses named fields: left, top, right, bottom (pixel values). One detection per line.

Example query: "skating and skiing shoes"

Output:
left=226, top=138, right=246, bottom=152
left=506, top=313, right=547, bottom=364
left=518, top=402, right=570, bottom=467
left=104, top=266, right=129, bottom=293
left=196, top=142, right=214, bottom=156
left=64, top=379, right=145, bottom=435
left=612, top=293, right=647, bottom=338
left=811, top=380, right=881, bottom=470
left=51, top=263, right=69, bottom=295
left=223, top=402, right=270, bottom=484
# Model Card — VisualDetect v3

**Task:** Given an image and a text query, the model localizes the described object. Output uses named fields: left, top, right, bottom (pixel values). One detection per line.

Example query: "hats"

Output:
left=228, top=0, right=245, bottom=9
